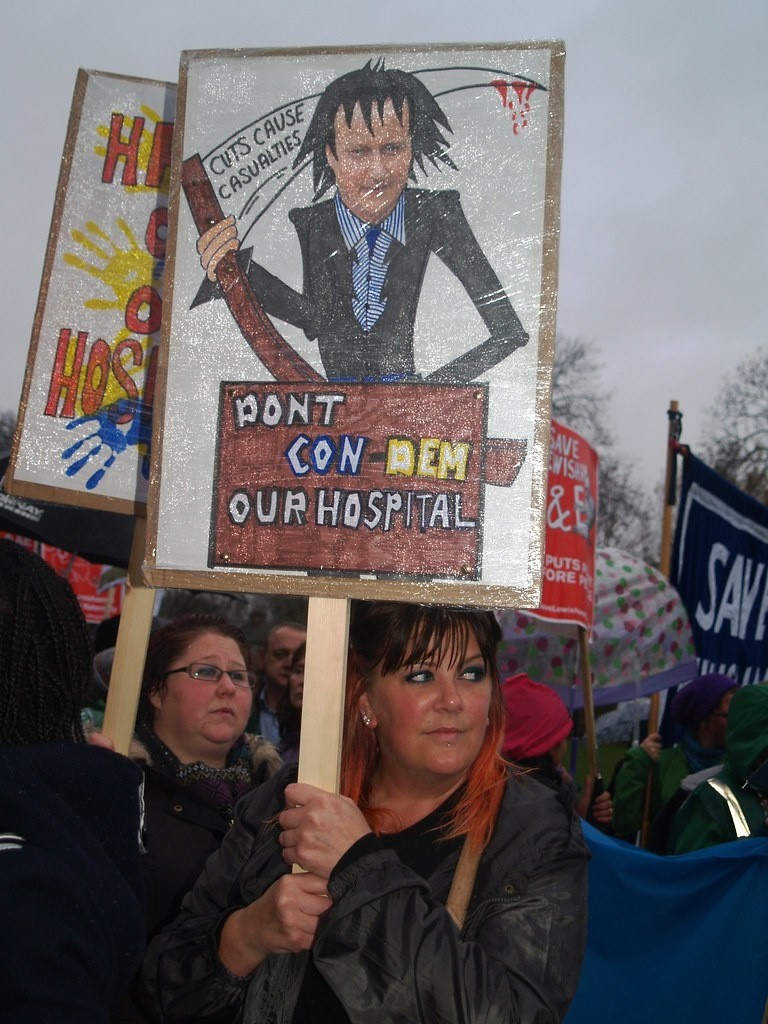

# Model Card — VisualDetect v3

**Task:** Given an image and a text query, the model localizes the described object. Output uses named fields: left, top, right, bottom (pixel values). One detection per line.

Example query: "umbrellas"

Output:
left=0, top=408, right=133, bottom=583
left=492, top=547, right=698, bottom=797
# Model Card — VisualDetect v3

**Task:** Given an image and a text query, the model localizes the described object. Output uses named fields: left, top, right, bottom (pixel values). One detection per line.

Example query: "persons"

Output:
left=153, top=599, right=591, bottom=1024
left=0, top=539, right=147, bottom=1024
left=675, top=684, right=768, bottom=853
left=494, top=671, right=614, bottom=831
left=611, top=673, right=741, bottom=856
left=111, top=611, right=286, bottom=1023
left=69, top=613, right=306, bottom=854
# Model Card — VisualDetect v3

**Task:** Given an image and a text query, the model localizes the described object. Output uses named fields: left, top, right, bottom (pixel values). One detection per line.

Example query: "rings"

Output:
left=610, top=808, right=613, bottom=814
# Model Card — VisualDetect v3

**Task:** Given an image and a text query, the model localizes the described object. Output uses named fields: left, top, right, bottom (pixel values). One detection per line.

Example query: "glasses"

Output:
left=162, top=661, right=260, bottom=689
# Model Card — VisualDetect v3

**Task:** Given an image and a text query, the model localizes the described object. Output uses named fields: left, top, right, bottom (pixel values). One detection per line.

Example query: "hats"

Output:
left=499, top=670, right=574, bottom=761
left=670, top=673, right=740, bottom=730
